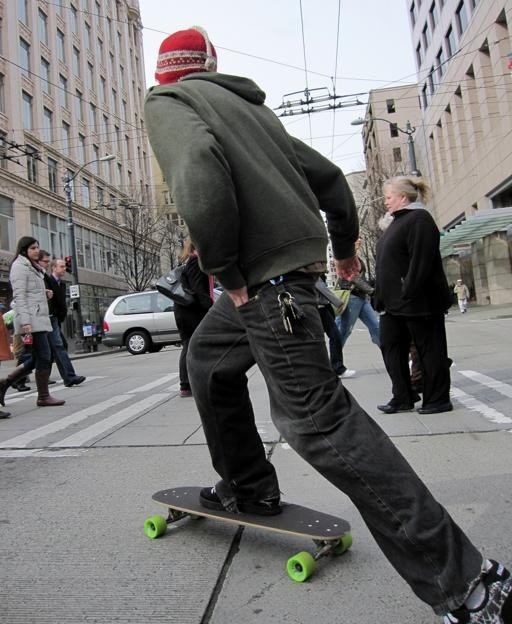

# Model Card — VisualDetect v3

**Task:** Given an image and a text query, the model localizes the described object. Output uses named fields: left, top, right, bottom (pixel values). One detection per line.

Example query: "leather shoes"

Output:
left=417, top=401, right=453, bottom=414
left=377, top=405, right=414, bottom=413
left=0, top=363, right=85, bottom=417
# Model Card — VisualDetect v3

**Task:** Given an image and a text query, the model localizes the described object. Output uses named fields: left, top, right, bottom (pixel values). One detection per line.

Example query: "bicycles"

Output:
left=82, top=336, right=97, bottom=353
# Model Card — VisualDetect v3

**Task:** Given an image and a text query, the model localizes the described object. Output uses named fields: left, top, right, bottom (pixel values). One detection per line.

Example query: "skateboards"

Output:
left=142, top=487, right=351, bottom=582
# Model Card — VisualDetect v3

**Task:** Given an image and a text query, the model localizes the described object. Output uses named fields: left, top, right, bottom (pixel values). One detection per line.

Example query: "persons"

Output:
left=369, top=176, right=453, bottom=415
left=313, top=272, right=356, bottom=378
left=334, top=230, right=414, bottom=364
left=1, top=236, right=85, bottom=420
left=143, top=26, right=511, bottom=624
left=453, top=277, right=472, bottom=313
left=376, top=212, right=453, bottom=395
left=169, top=233, right=215, bottom=399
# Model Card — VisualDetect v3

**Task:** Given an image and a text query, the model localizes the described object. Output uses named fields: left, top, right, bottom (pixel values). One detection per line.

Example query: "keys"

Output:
left=277, top=291, right=307, bottom=336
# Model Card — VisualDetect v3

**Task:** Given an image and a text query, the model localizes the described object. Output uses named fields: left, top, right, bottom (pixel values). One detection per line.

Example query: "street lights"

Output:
left=57, top=154, right=117, bottom=359
left=348, top=117, right=418, bottom=172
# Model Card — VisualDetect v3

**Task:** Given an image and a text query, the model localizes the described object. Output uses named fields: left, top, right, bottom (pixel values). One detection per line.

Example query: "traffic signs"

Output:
left=68, top=285, right=80, bottom=300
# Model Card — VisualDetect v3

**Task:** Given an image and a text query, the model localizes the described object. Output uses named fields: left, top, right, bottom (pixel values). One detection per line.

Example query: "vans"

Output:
left=102, top=289, right=223, bottom=356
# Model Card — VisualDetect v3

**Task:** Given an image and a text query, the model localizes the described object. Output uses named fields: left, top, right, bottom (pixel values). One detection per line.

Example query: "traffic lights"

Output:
left=64, top=255, right=71, bottom=273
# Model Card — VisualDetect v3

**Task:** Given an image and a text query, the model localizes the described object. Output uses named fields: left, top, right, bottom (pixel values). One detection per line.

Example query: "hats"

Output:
left=155, top=26, right=217, bottom=85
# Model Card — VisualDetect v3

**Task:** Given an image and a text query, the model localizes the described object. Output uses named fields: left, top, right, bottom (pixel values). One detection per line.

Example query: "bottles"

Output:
left=25, top=330, right=34, bottom=344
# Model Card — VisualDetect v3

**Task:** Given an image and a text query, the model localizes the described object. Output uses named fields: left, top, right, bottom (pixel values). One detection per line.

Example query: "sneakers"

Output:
left=337, top=370, right=356, bottom=378
left=445, top=558, right=511, bottom=623
left=179, top=390, right=192, bottom=397
left=199, top=487, right=280, bottom=514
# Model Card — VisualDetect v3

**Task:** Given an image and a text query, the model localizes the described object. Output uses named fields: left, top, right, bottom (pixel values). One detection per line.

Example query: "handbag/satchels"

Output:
left=331, top=290, right=351, bottom=314
left=155, top=263, right=193, bottom=306
